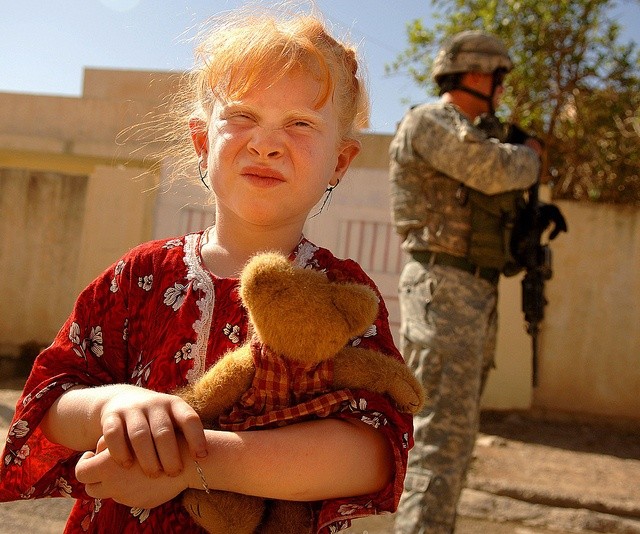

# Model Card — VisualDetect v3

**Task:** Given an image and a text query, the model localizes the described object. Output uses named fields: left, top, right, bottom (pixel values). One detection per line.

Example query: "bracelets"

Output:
left=192, top=452, right=210, bottom=494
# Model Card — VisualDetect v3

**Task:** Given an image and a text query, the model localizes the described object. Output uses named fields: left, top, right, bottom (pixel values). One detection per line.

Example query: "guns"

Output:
left=503, top=124, right=566, bottom=387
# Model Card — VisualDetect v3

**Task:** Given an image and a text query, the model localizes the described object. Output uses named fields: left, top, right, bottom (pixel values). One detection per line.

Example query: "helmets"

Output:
left=432, top=27, right=516, bottom=77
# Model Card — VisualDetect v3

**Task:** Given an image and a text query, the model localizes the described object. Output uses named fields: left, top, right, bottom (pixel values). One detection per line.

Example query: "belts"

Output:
left=408, top=252, right=500, bottom=286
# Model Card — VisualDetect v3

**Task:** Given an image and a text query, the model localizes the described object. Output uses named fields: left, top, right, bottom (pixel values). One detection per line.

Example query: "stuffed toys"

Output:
left=178, top=250, right=424, bottom=534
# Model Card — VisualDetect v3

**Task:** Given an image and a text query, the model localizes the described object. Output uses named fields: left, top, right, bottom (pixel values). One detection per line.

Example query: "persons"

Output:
left=1, top=0, right=415, bottom=534
left=387, top=28, right=543, bottom=534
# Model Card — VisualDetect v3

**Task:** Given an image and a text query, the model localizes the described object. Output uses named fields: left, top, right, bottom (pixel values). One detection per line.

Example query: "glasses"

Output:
left=491, top=69, right=506, bottom=86
